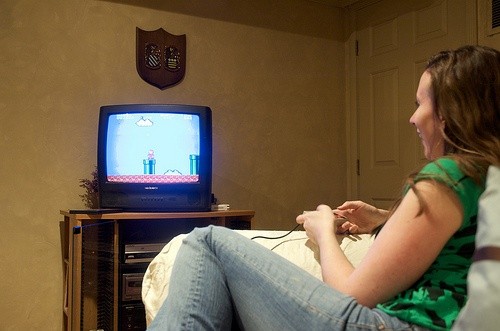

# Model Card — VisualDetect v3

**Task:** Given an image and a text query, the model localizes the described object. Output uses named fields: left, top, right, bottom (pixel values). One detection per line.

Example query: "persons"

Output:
left=147, top=45, right=500, bottom=331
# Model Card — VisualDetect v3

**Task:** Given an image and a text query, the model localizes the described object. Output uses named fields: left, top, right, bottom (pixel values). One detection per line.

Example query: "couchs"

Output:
left=141, top=166, right=500, bottom=331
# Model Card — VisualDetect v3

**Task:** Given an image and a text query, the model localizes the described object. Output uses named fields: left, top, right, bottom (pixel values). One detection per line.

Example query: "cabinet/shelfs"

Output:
left=59, top=210, right=255, bottom=331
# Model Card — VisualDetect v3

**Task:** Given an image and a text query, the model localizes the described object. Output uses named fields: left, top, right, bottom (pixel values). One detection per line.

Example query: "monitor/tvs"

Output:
left=97, top=104, right=213, bottom=212
